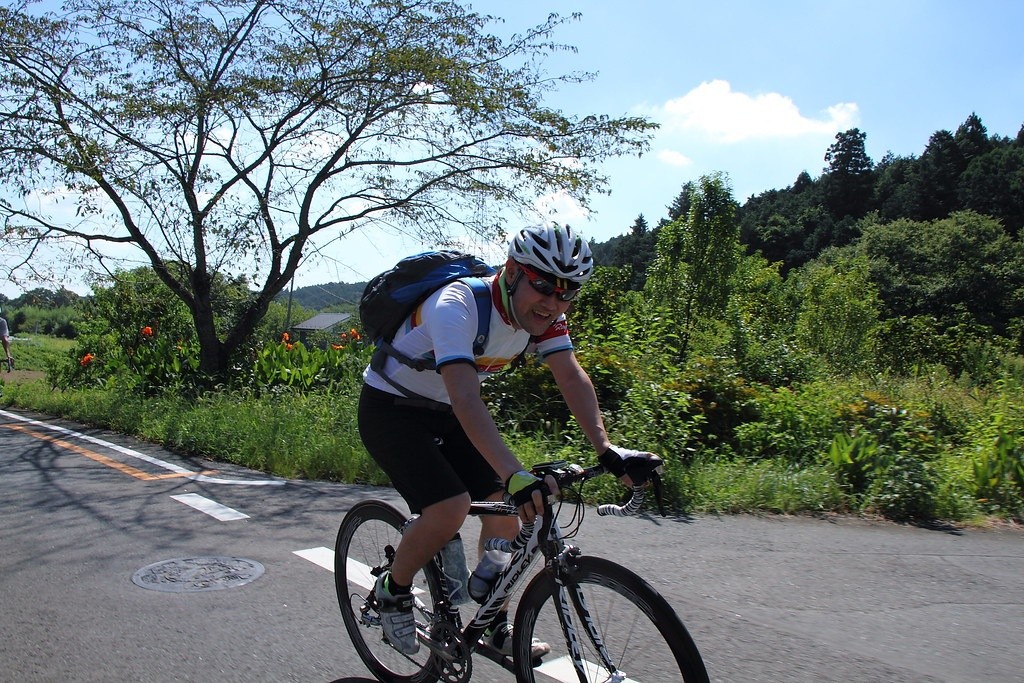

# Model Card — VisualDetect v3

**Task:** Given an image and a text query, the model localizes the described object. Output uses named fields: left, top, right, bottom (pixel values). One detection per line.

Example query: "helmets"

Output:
left=508, top=223, right=594, bottom=284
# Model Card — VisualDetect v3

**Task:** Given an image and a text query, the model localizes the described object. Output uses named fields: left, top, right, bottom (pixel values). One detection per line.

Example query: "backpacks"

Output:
left=359, top=252, right=497, bottom=374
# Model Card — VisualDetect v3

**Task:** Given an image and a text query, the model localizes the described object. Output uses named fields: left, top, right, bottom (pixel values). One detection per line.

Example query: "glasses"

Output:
left=517, top=261, right=581, bottom=302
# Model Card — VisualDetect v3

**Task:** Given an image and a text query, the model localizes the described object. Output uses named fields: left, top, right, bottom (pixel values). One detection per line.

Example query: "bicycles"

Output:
left=333, top=459, right=709, bottom=683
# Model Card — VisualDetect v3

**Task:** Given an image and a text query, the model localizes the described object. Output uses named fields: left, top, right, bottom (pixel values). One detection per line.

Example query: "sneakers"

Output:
left=376, top=571, right=420, bottom=655
left=483, top=624, right=549, bottom=657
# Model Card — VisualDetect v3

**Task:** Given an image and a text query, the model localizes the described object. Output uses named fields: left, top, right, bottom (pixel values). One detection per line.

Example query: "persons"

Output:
left=357, top=221, right=663, bottom=660
left=0, top=317, right=14, bottom=397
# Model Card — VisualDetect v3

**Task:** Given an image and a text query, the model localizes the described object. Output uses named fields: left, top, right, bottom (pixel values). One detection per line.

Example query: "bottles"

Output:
left=439, top=532, right=474, bottom=605
left=467, top=549, right=512, bottom=598
